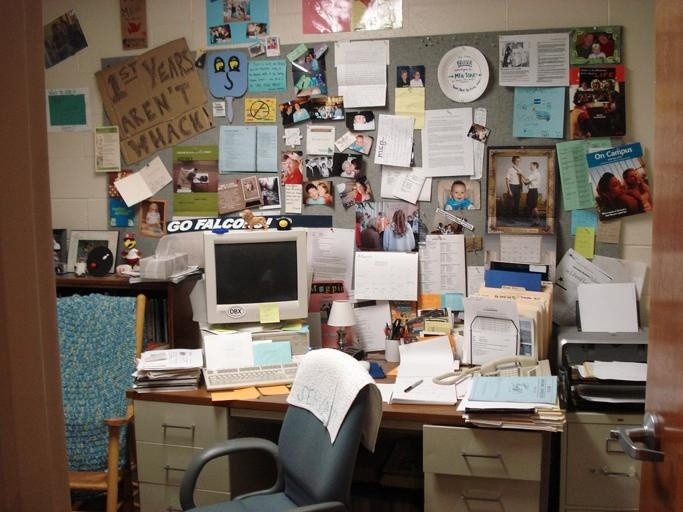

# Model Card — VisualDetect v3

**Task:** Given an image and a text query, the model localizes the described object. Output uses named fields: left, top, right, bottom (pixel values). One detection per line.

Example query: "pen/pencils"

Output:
left=384, top=322, right=402, bottom=340
left=404, top=379, right=423, bottom=393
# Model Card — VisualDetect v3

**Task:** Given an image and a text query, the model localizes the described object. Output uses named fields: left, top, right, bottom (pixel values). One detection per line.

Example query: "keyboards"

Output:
left=202, top=363, right=299, bottom=391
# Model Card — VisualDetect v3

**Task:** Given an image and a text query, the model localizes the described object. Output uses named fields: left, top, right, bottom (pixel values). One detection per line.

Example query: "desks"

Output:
left=126, top=348, right=566, bottom=512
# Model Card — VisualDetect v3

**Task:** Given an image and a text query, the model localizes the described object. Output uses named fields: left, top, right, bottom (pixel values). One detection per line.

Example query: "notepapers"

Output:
left=257, top=385, right=290, bottom=396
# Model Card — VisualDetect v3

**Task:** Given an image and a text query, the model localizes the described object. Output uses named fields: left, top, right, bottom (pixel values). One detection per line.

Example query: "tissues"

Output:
left=139, top=233, right=188, bottom=280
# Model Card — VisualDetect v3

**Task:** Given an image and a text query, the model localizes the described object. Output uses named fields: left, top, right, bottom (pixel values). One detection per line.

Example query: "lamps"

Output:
left=327, top=300, right=358, bottom=351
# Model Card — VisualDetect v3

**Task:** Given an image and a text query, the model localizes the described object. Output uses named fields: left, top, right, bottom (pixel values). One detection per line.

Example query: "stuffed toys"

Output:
left=238, top=209, right=268, bottom=231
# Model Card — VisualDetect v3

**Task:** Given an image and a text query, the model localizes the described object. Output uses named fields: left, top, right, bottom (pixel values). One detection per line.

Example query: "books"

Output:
left=390, top=260, right=568, bottom=433
left=131, top=347, right=203, bottom=392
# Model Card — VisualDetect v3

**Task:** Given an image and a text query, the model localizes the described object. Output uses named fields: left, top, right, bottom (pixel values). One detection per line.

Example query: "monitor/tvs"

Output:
left=203, top=231, right=309, bottom=332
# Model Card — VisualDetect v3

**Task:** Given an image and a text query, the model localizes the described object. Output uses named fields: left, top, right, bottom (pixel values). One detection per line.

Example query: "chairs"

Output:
left=55, top=292, right=147, bottom=512
left=178, top=347, right=384, bottom=512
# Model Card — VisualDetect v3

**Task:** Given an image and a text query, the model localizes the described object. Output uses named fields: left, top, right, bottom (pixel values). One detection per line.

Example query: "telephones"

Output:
left=480, top=355, right=552, bottom=377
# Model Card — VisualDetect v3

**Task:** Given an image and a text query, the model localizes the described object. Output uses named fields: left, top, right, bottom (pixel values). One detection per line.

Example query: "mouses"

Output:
left=360, top=360, right=370, bottom=372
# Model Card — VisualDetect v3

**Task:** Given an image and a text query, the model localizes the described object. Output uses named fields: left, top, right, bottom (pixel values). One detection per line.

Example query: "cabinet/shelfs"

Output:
left=56, top=271, right=204, bottom=348
left=559, top=407, right=645, bottom=512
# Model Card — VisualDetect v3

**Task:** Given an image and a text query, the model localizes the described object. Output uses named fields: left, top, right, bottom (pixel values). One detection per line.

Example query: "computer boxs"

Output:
left=198, top=320, right=310, bottom=368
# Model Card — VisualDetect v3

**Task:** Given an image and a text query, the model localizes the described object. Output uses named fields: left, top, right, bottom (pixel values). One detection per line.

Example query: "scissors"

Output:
left=391, top=319, right=405, bottom=339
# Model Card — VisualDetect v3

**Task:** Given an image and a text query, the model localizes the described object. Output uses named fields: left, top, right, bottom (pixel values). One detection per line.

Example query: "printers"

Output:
left=556, top=300, right=648, bottom=413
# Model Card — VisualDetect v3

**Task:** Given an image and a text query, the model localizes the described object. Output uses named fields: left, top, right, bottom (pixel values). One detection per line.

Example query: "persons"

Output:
left=503, top=156, right=523, bottom=224
left=350, top=135, right=366, bottom=153
left=141, top=203, right=162, bottom=233
left=409, top=70, right=423, bottom=87
left=597, top=34, right=615, bottom=57
left=514, top=44, right=528, bottom=67
left=502, top=43, right=516, bottom=68
left=282, top=53, right=342, bottom=125
left=261, top=187, right=268, bottom=205
left=210, top=0, right=277, bottom=51
left=443, top=180, right=475, bottom=212
left=435, top=222, right=457, bottom=236
left=578, top=34, right=593, bottom=59
left=516, top=161, right=541, bottom=223
left=397, top=69, right=410, bottom=88
left=280, top=150, right=419, bottom=252
left=346, top=111, right=375, bottom=130
left=571, top=79, right=624, bottom=140
left=587, top=43, right=608, bottom=58
left=594, top=173, right=650, bottom=216
left=624, top=167, right=650, bottom=202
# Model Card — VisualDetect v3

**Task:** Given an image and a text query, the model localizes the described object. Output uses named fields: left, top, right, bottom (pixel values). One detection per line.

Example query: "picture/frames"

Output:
left=487, top=146, right=557, bottom=235
